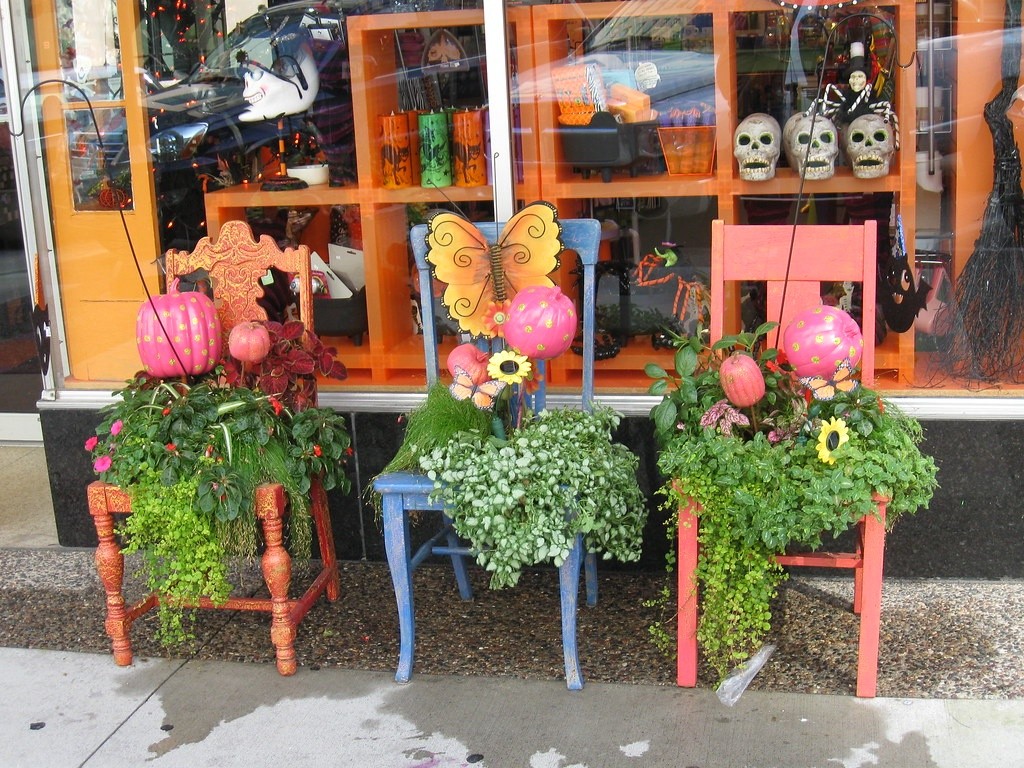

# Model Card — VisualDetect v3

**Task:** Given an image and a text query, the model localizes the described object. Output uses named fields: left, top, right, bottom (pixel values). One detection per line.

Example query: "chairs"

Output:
left=373, top=218, right=602, bottom=691
left=88, top=220, right=340, bottom=674
left=671, top=219, right=892, bottom=697
left=271, top=26, right=317, bottom=72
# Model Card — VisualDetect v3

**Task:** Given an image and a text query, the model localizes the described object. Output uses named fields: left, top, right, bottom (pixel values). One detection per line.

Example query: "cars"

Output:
left=100, top=0, right=472, bottom=194
left=508, top=0, right=794, bottom=172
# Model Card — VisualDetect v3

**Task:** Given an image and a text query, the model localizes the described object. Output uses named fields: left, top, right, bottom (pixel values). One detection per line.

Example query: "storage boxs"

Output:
left=659, top=126, right=716, bottom=177
left=557, top=112, right=657, bottom=183
left=295, top=295, right=368, bottom=347
left=551, top=63, right=657, bottom=122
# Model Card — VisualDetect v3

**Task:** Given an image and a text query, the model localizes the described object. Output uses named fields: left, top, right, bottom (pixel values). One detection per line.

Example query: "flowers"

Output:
left=645, top=326, right=942, bottom=691
left=487, top=350, right=533, bottom=417
left=86, top=322, right=352, bottom=662
left=816, top=416, right=850, bottom=465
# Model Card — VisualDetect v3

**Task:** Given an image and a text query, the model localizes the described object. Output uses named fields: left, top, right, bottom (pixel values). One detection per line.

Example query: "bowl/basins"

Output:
left=287, top=163, right=330, bottom=185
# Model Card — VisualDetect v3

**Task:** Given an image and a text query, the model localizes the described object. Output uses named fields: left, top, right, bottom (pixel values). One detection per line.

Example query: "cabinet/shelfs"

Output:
left=530, top=1, right=916, bottom=397
left=207, top=0, right=551, bottom=390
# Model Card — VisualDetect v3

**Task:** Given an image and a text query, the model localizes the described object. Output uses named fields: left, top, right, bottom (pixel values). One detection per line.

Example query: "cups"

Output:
left=376, top=107, right=492, bottom=187
